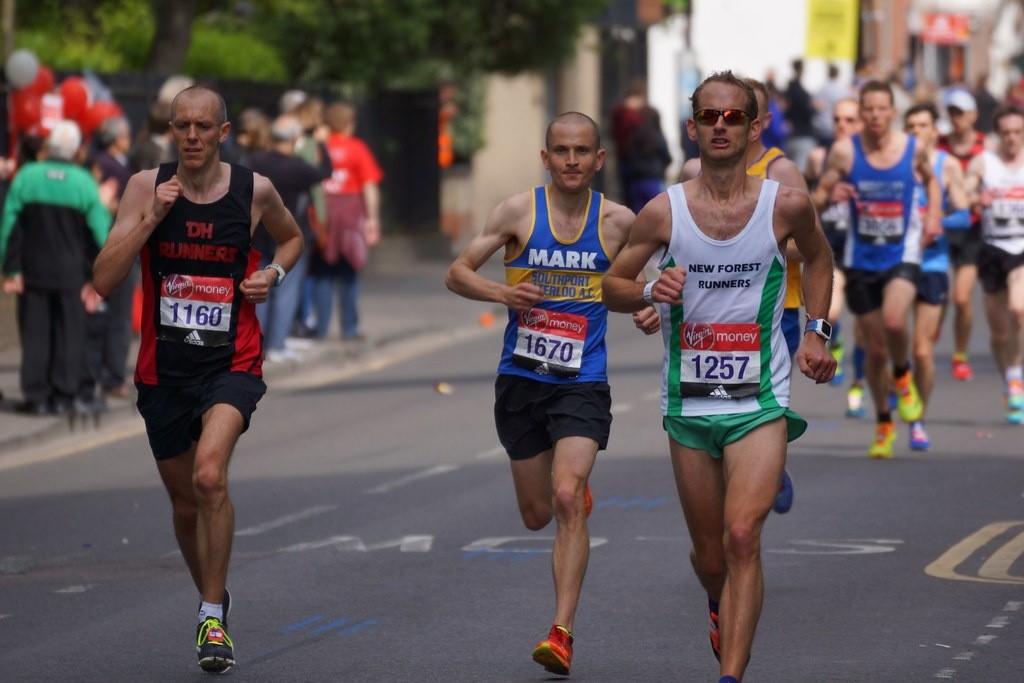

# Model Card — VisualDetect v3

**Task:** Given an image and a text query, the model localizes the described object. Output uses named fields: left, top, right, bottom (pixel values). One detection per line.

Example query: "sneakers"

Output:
left=708, top=593, right=721, bottom=663
left=719, top=676, right=738, bottom=683
left=532, top=624, right=574, bottom=676
left=583, top=483, right=593, bottom=517
left=868, top=421, right=896, bottom=458
left=892, top=368, right=924, bottom=423
left=196, top=587, right=235, bottom=674
left=772, top=469, right=793, bottom=514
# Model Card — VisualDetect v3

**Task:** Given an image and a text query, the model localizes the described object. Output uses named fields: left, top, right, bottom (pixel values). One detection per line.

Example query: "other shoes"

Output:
left=951, top=352, right=972, bottom=381
left=1002, top=379, right=1024, bottom=424
left=846, top=385, right=865, bottom=417
left=909, top=421, right=931, bottom=451
left=827, top=336, right=846, bottom=387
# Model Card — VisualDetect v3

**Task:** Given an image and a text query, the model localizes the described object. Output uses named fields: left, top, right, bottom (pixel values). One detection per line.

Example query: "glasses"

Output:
left=693, top=109, right=750, bottom=126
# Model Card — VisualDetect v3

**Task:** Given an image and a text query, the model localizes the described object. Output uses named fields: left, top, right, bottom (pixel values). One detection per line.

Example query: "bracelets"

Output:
left=643, top=278, right=659, bottom=306
left=824, top=187, right=837, bottom=209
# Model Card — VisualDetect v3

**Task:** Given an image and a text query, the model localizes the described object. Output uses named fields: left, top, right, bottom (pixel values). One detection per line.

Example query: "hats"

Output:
left=942, top=87, right=976, bottom=113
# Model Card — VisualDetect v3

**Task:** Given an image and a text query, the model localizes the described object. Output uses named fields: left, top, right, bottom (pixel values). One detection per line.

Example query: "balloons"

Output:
left=5, top=50, right=120, bottom=142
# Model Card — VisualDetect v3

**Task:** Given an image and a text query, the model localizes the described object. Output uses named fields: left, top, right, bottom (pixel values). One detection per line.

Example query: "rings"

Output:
left=648, top=325, right=654, bottom=332
left=822, top=372, right=829, bottom=378
left=249, top=296, right=252, bottom=301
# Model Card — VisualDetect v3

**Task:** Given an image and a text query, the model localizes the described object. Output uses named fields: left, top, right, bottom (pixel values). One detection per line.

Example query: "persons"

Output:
left=0, top=73, right=382, bottom=417
left=444, top=112, right=663, bottom=676
left=602, top=70, right=838, bottom=683
left=93, top=85, right=304, bottom=676
left=613, top=56, right=1024, bottom=516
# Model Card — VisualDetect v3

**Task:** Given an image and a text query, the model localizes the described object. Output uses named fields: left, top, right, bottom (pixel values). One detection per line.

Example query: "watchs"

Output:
left=264, top=263, right=286, bottom=288
left=803, top=318, right=833, bottom=342
